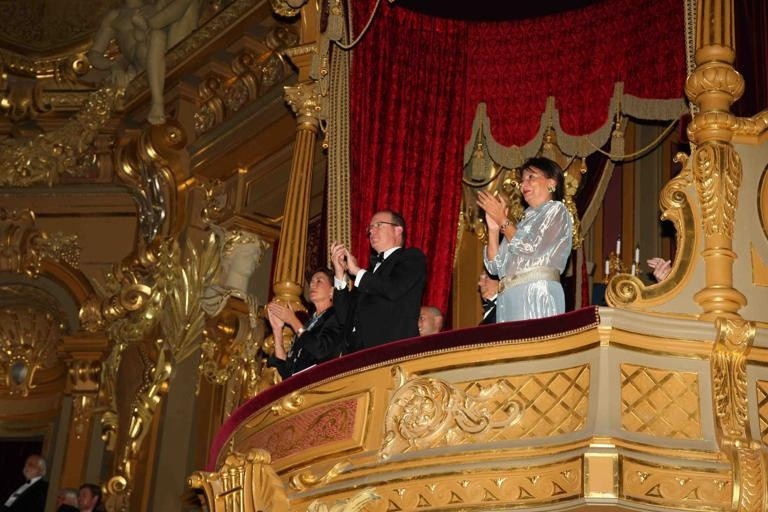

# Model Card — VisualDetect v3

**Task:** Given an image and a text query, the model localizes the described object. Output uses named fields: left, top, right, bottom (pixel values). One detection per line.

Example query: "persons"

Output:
left=264, top=210, right=444, bottom=382
left=55, top=483, right=108, bottom=512
left=0, top=454, right=49, bottom=512
left=477, top=156, right=573, bottom=325
left=646, top=258, right=672, bottom=283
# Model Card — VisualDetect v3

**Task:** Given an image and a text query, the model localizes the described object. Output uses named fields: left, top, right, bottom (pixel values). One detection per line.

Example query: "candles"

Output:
left=604, top=236, right=640, bottom=277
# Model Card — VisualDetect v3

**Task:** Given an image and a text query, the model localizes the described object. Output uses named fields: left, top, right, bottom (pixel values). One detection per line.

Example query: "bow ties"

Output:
left=483, top=298, right=495, bottom=312
left=370, top=251, right=384, bottom=267
left=25, top=479, right=30, bottom=483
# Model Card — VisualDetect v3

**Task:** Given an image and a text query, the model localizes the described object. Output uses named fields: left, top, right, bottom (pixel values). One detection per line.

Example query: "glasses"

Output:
left=369, top=221, right=398, bottom=232
